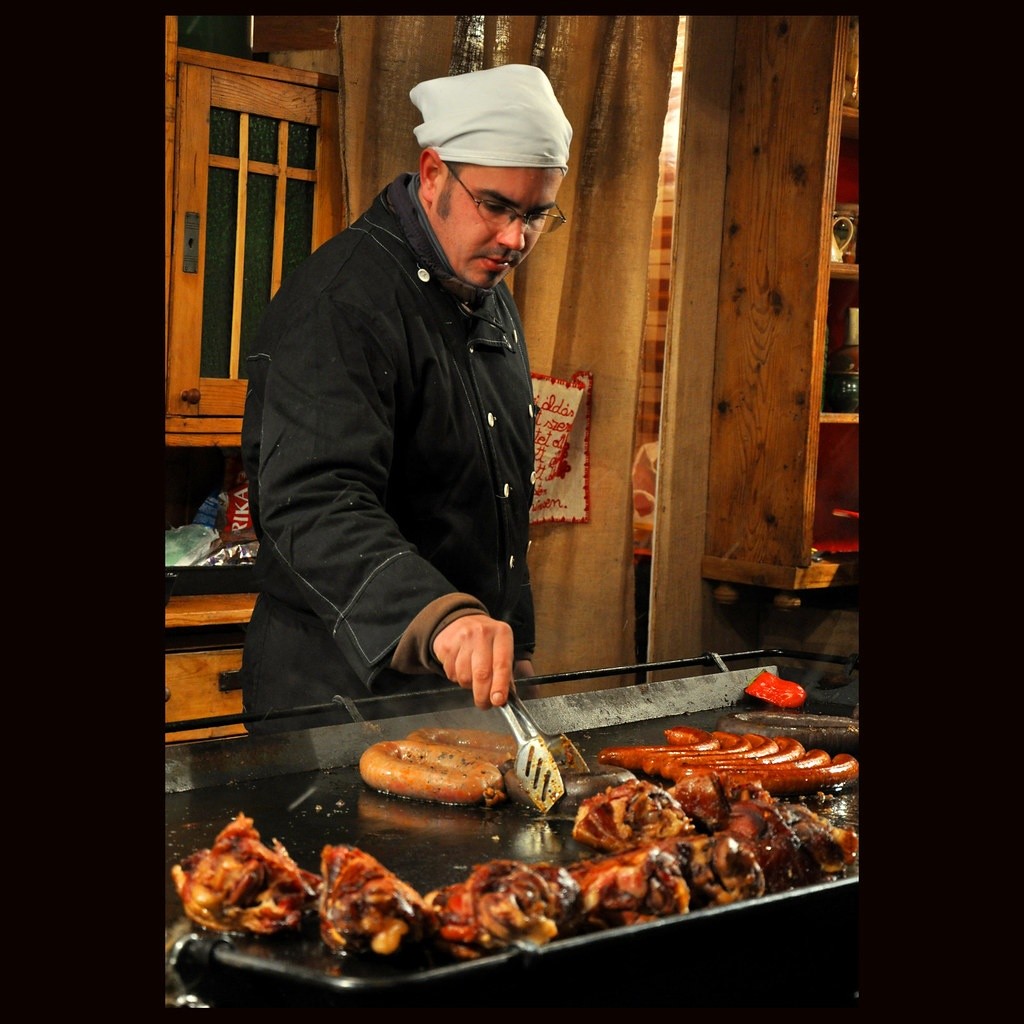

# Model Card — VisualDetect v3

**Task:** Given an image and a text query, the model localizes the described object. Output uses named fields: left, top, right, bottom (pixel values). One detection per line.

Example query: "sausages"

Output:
left=599, top=726, right=860, bottom=795
left=358, top=728, right=535, bottom=804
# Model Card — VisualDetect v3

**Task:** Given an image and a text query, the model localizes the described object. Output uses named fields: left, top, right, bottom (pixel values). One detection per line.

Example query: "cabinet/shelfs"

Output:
left=165, top=14, right=345, bottom=449
left=702, top=17, right=859, bottom=608
left=164, top=593, right=259, bottom=744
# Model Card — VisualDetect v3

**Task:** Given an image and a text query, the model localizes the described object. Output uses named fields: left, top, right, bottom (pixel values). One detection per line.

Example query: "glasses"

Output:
left=444, top=162, right=567, bottom=233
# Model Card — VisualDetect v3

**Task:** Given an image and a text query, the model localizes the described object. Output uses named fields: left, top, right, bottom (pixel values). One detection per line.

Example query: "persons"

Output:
left=241, top=64, right=574, bottom=713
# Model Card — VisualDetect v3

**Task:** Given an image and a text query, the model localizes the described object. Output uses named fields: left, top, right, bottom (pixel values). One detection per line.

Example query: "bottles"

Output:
left=838, top=306, right=859, bottom=370
left=823, top=371, right=859, bottom=412
left=834, top=210, right=858, bottom=264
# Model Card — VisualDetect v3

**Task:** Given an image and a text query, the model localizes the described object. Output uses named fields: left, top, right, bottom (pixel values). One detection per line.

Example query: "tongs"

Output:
left=498, top=687, right=591, bottom=814
